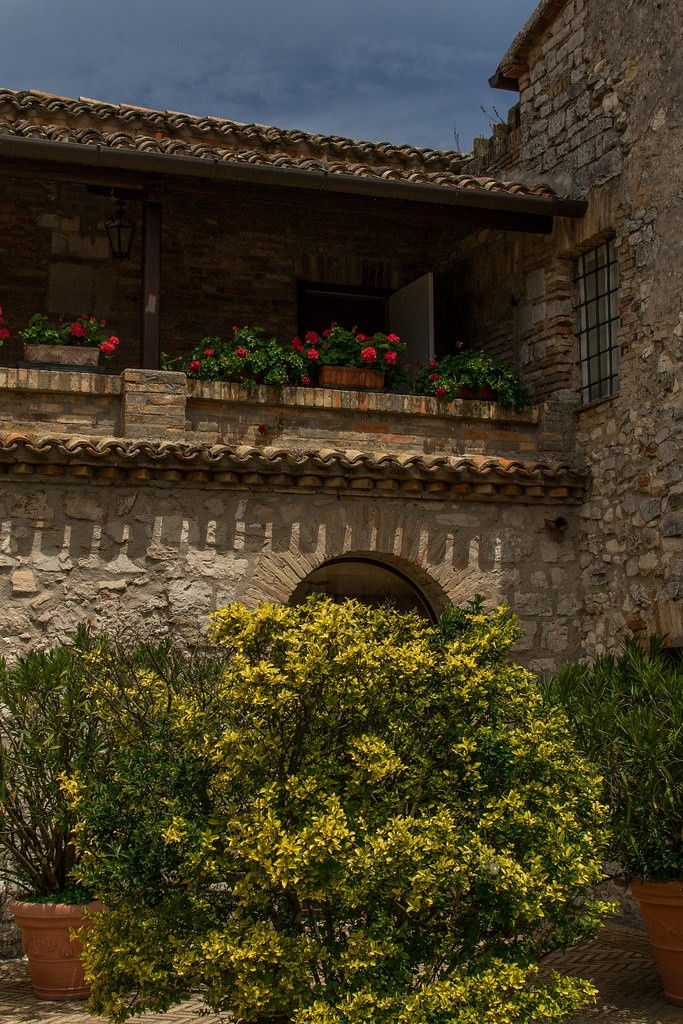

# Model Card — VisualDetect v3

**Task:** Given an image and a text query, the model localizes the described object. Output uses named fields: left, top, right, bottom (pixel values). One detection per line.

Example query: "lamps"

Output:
left=104, top=187, right=147, bottom=262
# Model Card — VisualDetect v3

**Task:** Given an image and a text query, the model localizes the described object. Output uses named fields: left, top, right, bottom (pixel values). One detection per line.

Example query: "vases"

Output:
left=453, top=383, right=500, bottom=404
left=24, top=344, right=99, bottom=367
left=317, top=365, right=384, bottom=390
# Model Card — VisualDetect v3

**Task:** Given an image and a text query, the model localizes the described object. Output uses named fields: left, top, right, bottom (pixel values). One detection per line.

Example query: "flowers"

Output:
left=182, top=317, right=410, bottom=391
left=19, top=313, right=120, bottom=355
left=0, top=307, right=9, bottom=348
left=411, top=339, right=534, bottom=414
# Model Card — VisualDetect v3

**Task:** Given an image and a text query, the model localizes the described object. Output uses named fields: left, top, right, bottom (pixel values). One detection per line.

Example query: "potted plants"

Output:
left=534, top=631, right=683, bottom=1008
left=1, top=618, right=143, bottom=999
